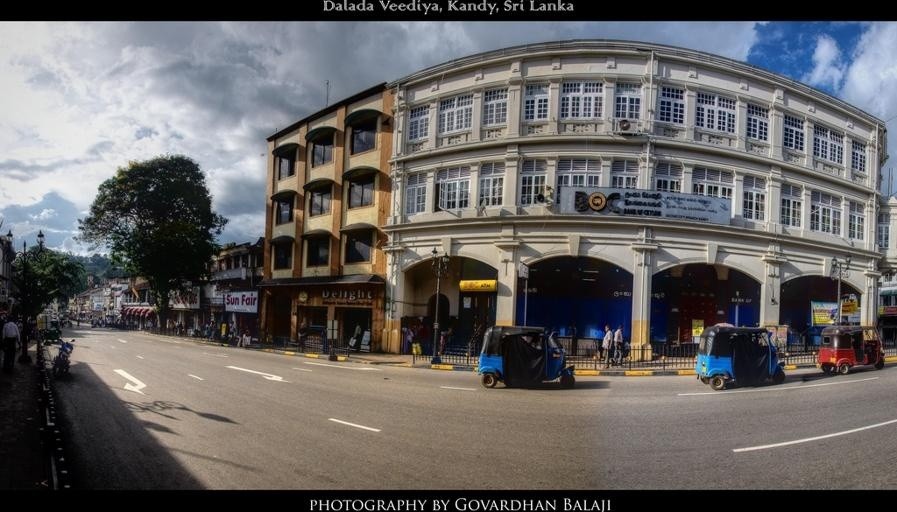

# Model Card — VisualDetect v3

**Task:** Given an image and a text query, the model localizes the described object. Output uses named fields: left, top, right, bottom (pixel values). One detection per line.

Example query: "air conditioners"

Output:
left=610, top=117, right=639, bottom=135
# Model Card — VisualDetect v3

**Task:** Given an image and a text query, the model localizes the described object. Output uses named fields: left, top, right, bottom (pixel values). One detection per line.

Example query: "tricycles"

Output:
left=477, top=325, right=576, bottom=388
left=818, top=325, right=886, bottom=376
left=693, top=322, right=786, bottom=390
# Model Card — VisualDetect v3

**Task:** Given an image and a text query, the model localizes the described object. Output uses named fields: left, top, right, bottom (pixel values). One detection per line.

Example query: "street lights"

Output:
left=430, top=248, right=449, bottom=364
left=3, top=230, right=47, bottom=365
left=829, top=255, right=852, bottom=325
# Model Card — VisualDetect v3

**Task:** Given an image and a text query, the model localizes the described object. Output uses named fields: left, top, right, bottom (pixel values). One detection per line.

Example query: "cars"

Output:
left=45, top=309, right=86, bottom=321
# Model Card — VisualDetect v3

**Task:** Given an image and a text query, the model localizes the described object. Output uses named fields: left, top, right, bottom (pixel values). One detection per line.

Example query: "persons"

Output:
left=2, top=315, right=23, bottom=369
left=297, top=323, right=307, bottom=353
left=146, top=316, right=251, bottom=348
left=602, top=325, right=614, bottom=369
left=612, top=324, right=626, bottom=367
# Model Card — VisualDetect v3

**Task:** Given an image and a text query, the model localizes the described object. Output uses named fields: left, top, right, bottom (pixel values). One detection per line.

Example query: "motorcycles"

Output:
left=92, top=318, right=97, bottom=328
left=175, top=320, right=184, bottom=336
left=98, top=317, right=102, bottom=327
left=52, top=337, right=76, bottom=381
